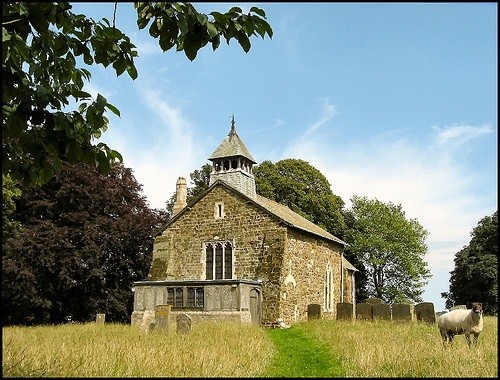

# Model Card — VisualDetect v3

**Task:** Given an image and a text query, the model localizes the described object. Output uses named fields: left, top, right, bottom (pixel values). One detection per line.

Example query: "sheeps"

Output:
left=437, top=301, right=484, bottom=350
